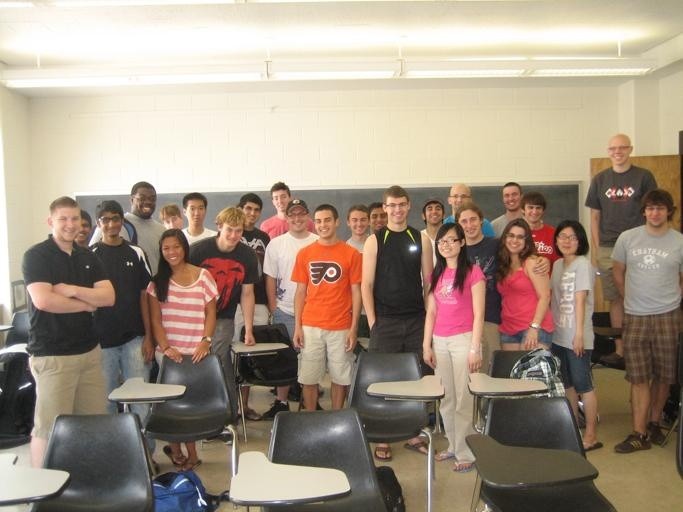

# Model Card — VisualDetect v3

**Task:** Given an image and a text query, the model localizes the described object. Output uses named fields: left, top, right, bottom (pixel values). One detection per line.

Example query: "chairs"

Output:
left=4, top=311, right=30, bottom=345
left=141, top=353, right=239, bottom=508
left=263, top=410, right=386, bottom=512
left=347, top=351, right=436, bottom=512
left=483, top=398, right=623, bottom=512
left=240, top=323, right=298, bottom=444
left=491, top=312, right=625, bottom=378
left=32, top=414, right=155, bottom=512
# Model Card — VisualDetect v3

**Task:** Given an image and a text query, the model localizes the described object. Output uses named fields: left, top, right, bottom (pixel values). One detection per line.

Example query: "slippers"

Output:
left=583, top=441, right=603, bottom=451
left=163, top=445, right=202, bottom=472
left=453, top=461, right=473, bottom=472
left=374, top=446, right=392, bottom=462
left=435, top=450, right=456, bottom=461
left=404, top=442, right=437, bottom=455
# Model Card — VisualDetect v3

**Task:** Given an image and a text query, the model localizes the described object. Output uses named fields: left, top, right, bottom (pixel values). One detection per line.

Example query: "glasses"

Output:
left=558, top=233, right=578, bottom=241
left=99, top=217, right=119, bottom=223
left=435, top=238, right=462, bottom=243
left=384, top=203, right=407, bottom=208
left=289, top=212, right=307, bottom=217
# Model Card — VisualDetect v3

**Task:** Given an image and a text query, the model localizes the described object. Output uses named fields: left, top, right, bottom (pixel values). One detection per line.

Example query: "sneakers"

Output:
left=615, top=433, right=651, bottom=453
left=648, top=421, right=665, bottom=444
left=243, top=384, right=323, bottom=419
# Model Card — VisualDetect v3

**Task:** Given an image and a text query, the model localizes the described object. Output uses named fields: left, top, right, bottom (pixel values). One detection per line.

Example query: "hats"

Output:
left=286, top=199, right=308, bottom=212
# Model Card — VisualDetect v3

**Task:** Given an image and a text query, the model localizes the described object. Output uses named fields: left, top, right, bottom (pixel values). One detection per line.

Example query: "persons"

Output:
left=584, top=134, right=658, bottom=367
left=420, top=183, right=604, bottom=472
left=74, top=179, right=258, bottom=477
left=236, top=180, right=437, bottom=461
left=611, top=190, right=683, bottom=454
left=22, top=196, right=116, bottom=469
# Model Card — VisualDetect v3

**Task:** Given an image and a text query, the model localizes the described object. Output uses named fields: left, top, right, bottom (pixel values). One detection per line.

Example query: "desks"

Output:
left=230, top=339, right=285, bottom=442
left=366, top=375, right=445, bottom=477
left=467, top=370, right=548, bottom=433
left=230, top=451, right=351, bottom=507
left=0, top=450, right=69, bottom=509
left=109, top=377, right=187, bottom=428
left=463, top=435, right=599, bottom=488
